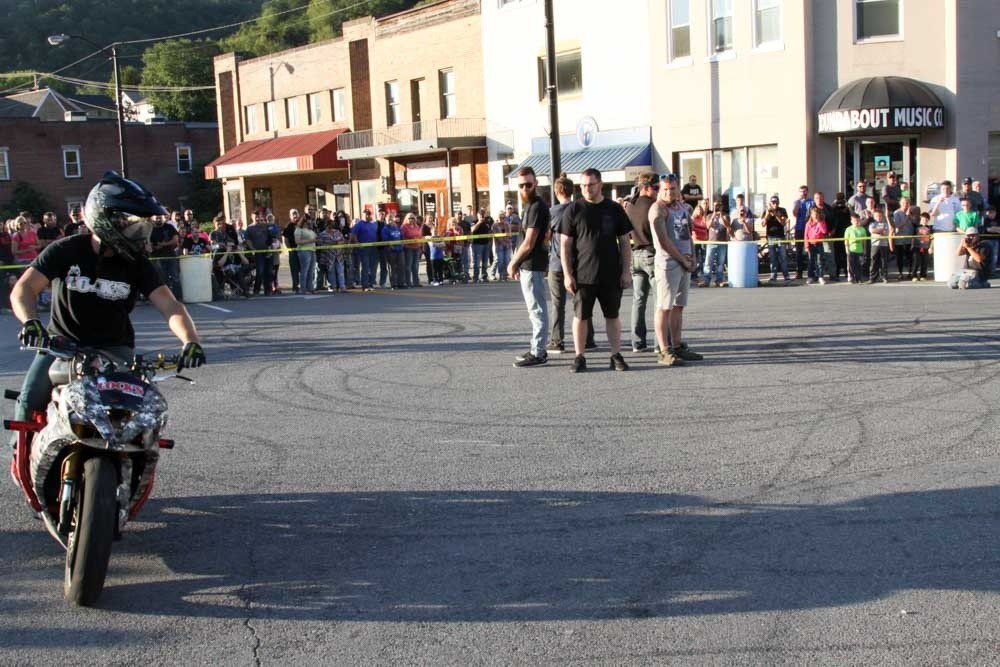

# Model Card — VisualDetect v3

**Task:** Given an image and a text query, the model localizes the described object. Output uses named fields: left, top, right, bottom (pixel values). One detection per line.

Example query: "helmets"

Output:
left=82, top=171, right=167, bottom=265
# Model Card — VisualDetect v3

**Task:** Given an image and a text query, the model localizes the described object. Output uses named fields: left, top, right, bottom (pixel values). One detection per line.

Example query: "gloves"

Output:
left=177, top=342, right=207, bottom=373
left=18, top=319, right=50, bottom=355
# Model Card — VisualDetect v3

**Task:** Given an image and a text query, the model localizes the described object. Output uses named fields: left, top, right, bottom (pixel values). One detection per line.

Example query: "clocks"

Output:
left=576, top=117, right=598, bottom=148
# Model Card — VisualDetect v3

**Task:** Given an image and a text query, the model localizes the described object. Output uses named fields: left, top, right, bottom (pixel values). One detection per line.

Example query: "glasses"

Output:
left=660, top=174, right=676, bottom=180
left=19, top=222, right=27, bottom=225
left=649, top=183, right=659, bottom=191
left=519, top=183, right=532, bottom=188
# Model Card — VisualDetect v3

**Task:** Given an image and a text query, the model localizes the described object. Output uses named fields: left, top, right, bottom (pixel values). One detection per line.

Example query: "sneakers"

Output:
left=673, top=343, right=703, bottom=360
left=657, top=349, right=684, bottom=365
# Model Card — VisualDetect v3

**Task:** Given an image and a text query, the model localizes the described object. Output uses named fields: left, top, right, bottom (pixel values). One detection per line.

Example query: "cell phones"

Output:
left=715, top=204, right=720, bottom=212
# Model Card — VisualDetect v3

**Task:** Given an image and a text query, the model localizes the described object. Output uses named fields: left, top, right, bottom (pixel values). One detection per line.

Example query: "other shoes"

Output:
left=11, top=459, right=33, bottom=488
left=0, top=299, right=49, bottom=315
left=654, top=346, right=660, bottom=353
left=691, top=272, right=927, bottom=287
left=515, top=352, right=549, bottom=361
left=230, top=273, right=520, bottom=297
left=585, top=342, right=597, bottom=352
left=546, top=342, right=565, bottom=353
left=514, top=355, right=545, bottom=368
left=569, top=354, right=586, bottom=372
left=610, top=353, right=629, bottom=371
left=633, top=347, right=649, bottom=353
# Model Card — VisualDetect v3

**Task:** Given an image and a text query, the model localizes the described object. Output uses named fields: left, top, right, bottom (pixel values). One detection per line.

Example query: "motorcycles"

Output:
left=0, top=331, right=201, bottom=607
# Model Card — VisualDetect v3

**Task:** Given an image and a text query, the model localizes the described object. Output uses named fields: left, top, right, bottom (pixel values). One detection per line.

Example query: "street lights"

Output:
left=49, top=34, right=128, bottom=181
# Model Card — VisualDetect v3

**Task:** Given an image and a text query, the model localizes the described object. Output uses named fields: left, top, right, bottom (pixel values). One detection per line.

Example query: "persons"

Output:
left=625, top=171, right=660, bottom=351
left=505, top=166, right=550, bottom=368
left=0, top=202, right=523, bottom=315
left=656, top=174, right=704, bottom=365
left=10, top=169, right=206, bottom=528
left=676, top=171, right=1000, bottom=290
left=540, top=168, right=635, bottom=373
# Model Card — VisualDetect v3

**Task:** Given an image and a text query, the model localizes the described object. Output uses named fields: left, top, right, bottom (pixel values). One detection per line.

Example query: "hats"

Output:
left=962, top=177, right=972, bottom=184
left=966, top=227, right=979, bottom=236
left=887, top=171, right=896, bottom=179
left=506, top=204, right=513, bottom=209
left=770, top=196, right=778, bottom=202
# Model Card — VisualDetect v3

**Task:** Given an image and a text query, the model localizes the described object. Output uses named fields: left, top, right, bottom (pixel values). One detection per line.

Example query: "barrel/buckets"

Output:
left=933, top=233, right=967, bottom=282
left=180, top=258, right=212, bottom=302
left=728, top=243, right=758, bottom=288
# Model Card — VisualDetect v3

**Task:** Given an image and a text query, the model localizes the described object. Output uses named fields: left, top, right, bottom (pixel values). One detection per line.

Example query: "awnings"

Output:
left=508, top=142, right=652, bottom=192
left=205, top=127, right=351, bottom=179
left=818, top=75, right=947, bottom=137
